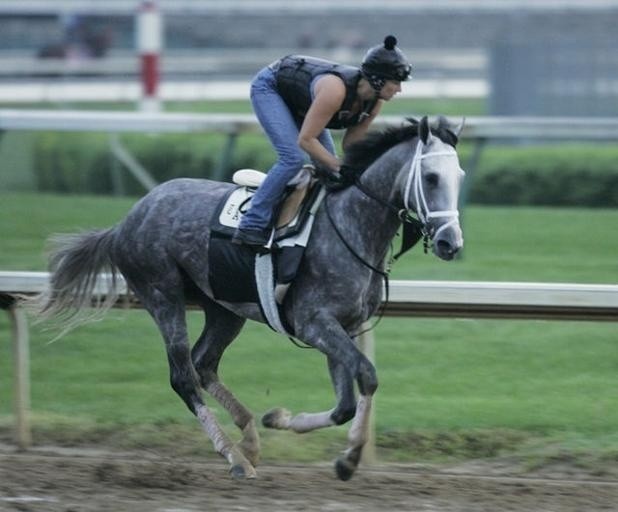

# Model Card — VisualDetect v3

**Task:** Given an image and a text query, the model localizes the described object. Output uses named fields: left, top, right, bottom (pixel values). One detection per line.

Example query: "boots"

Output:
left=232, top=229, right=268, bottom=245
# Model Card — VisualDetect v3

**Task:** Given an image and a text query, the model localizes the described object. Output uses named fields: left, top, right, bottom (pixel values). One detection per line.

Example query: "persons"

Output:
left=232, top=44, right=413, bottom=246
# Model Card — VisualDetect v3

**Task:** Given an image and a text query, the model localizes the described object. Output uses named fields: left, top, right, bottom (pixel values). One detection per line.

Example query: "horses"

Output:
left=11, top=115, right=466, bottom=482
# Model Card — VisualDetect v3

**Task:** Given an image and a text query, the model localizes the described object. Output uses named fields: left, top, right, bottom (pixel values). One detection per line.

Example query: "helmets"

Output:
left=361, top=43, right=413, bottom=83
